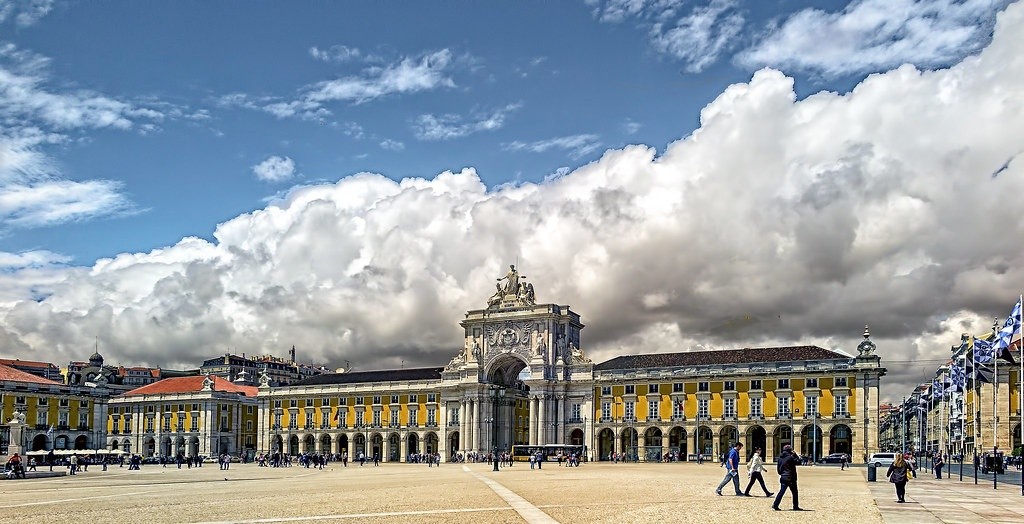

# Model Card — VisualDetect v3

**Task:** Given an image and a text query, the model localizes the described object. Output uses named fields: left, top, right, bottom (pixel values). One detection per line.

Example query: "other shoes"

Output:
left=744, top=492, right=752, bottom=497
left=766, top=492, right=774, bottom=497
left=736, top=492, right=744, bottom=496
left=897, top=499, right=905, bottom=503
left=793, top=507, right=803, bottom=511
left=716, top=489, right=723, bottom=496
left=772, top=504, right=781, bottom=511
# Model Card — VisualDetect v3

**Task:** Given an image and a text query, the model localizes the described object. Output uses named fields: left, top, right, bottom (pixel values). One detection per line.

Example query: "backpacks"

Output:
left=747, top=455, right=759, bottom=471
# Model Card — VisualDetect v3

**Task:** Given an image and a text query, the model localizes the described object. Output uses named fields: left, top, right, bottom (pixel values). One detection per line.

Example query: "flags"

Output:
left=920, top=297, right=1022, bottom=404
left=47, top=425, right=53, bottom=437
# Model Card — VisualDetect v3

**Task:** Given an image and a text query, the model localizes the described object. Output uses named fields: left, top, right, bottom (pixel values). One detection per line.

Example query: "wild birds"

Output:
left=224, top=477, right=228, bottom=481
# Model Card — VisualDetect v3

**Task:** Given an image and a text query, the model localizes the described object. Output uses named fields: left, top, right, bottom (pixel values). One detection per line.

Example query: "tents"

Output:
left=26, top=449, right=127, bottom=455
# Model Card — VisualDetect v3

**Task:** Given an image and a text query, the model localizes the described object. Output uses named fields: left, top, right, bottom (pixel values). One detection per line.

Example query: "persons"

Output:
left=886, top=453, right=912, bottom=503
left=716, top=442, right=744, bottom=496
left=608, top=451, right=684, bottom=464
left=556, top=334, right=565, bottom=356
left=452, top=449, right=513, bottom=471
left=408, top=453, right=441, bottom=467
left=696, top=449, right=727, bottom=468
left=772, top=445, right=803, bottom=511
left=903, top=450, right=981, bottom=479
left=517, top=450, right=581, bottom=470
left=219, top=449, right=380, bottom=470
left=536, top=334, right=545, bottom=356
left=567, top=342, right=582, bottom=356
left=799, top=453, right=851, bottom=470
left=744, top=447, right=774, bottom=497
left=7, top=452, right=203, bottom=479
left=487, top=265, right=535, bottom=307
left=448, top=350, right=465, bottom=366
left=471, top=339, right=478, bottom=361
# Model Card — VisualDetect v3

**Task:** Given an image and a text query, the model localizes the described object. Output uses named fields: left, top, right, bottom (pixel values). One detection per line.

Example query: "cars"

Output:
left=202, top=455, right=239, bottom=463
left=125, top=456, right=173, bottom=464
left=818, top=453, right=852, bottom=464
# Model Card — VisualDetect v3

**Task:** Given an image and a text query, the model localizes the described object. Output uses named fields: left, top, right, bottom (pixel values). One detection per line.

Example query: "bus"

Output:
left=511, top=444, right=588, bottom=462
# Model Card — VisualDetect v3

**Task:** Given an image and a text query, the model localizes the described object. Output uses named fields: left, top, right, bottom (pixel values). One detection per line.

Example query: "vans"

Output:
left=869, top=453, right=917, bottom=468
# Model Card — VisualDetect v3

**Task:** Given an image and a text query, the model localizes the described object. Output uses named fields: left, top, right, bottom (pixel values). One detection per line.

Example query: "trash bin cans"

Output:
left=868, top=463, right=876, bottom=482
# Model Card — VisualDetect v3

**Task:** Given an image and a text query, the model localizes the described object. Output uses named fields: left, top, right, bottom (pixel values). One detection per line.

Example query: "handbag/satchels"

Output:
left=906, top=468, right=912, bottom=480
